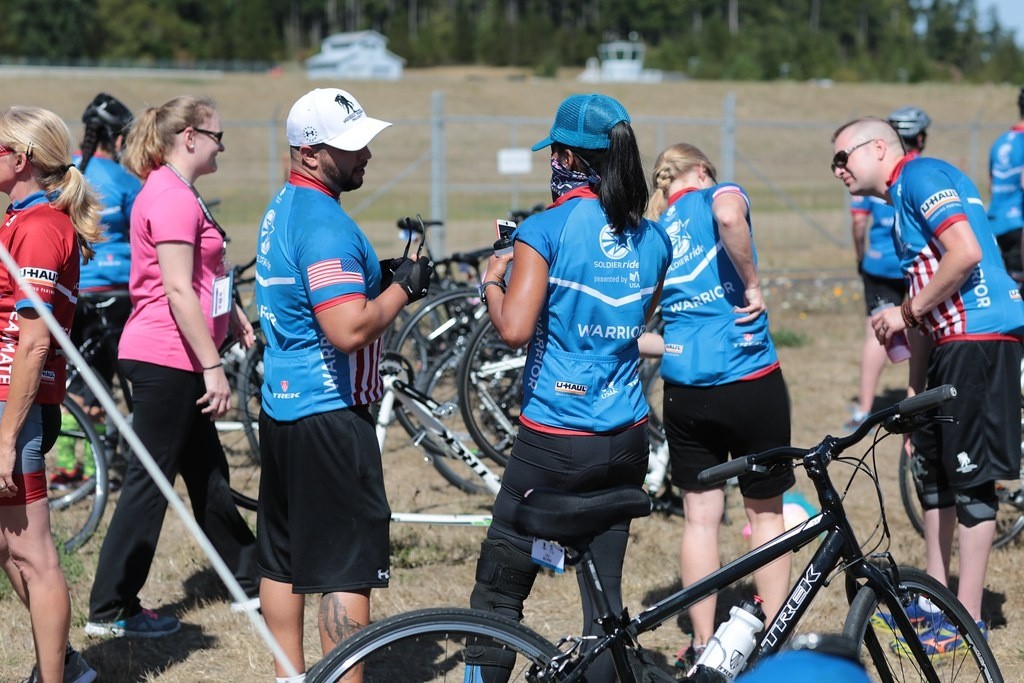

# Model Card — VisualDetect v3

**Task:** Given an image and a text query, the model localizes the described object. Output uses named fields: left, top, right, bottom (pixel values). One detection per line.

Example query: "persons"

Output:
left=638, top=142, right=795, bottom=678
left=830, top=116, right=1024, bottom=658
left=463, top=94, right=674, bottom=683
left=986, top=88, right=1024, bottom=297
left=0, top=107, right=105, bottom=683
left=49, top=93, right=143, bottom=491
left=843, top=107, right=933, bottom=431
left=255, top=88, right=434, bottom=683
left=82, top=93, right=262, bottom=639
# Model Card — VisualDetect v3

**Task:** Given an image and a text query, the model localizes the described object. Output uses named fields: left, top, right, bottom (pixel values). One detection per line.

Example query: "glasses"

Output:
left=831, top=138, right=875, bottom=174
left=175, top=126, right=224, bottom=144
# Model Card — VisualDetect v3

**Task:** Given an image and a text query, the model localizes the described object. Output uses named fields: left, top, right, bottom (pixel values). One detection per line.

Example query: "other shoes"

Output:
left=48, top=461, right=121, bottom=494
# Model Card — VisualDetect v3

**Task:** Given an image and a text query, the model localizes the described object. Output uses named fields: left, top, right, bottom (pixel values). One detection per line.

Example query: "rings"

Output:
left=882, top=324, right=888, bottom=331
left=0, top=483, right=7, bottom=490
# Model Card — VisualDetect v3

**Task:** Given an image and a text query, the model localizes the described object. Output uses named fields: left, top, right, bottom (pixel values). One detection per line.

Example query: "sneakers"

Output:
left=85, top=605, right=181, bottom=637
left=889, top=614, right=988, bottom=661
left=675, top=643, right=705, bottom=667
left=871, top=595, right=945, bottom=637
left=22, top=640, right=97, bottom=683
left=841, top=406, right=876, bottom=437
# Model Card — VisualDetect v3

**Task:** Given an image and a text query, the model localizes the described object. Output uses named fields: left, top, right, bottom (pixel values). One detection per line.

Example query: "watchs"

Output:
left=478, top=281, right=506, bottom=306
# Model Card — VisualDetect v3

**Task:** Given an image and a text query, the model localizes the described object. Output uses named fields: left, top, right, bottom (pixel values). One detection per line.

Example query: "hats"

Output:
left=531, top=93, right=631, bottom=152
left=286, top=88, right=392, bottom=152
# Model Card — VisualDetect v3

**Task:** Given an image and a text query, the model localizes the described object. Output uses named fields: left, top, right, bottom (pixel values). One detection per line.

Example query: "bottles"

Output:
left=871, top=293, right=912, bottom=364
left=493, top=230, right=515, bottom=287
left=686, top=596, right=768, bottom=683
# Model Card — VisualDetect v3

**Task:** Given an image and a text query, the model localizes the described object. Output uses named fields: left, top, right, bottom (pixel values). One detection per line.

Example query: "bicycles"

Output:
left=303, top=382, right=1004, bottom=683
left=45, top=204, right=685, bottom=555
left=899, top=437, right=1023, bottom=549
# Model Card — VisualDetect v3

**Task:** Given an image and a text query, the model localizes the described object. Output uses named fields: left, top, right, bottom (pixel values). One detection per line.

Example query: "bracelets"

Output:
left=901, top=297, right=924, bottom=330
left=203, top=363, right=223, bottom=370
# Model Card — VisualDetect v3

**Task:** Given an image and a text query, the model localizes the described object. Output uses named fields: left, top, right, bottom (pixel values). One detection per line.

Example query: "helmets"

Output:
left=84, top=93, right=136, bottom=128
left=888, top=106, right=931, bottom=136
